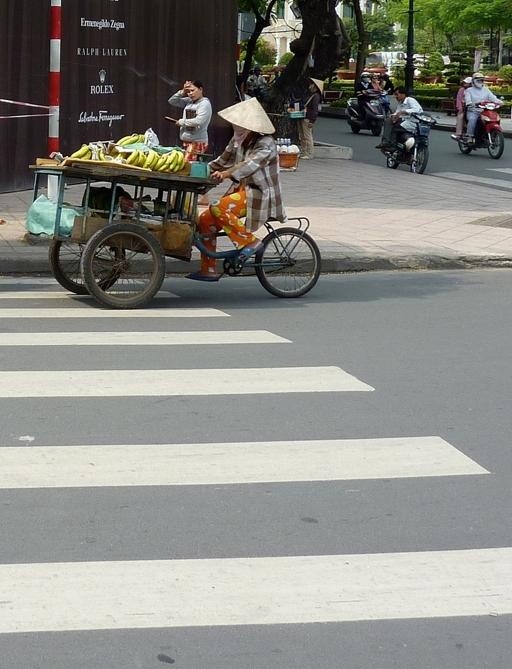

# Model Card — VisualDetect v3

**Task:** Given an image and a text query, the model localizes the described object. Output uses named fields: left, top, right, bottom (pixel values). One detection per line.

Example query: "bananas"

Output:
left=68, top=133, right=186, bottom=173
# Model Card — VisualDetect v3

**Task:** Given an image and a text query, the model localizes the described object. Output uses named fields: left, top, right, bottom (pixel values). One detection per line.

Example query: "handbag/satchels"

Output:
left=185, top=109, right=196, bottom=132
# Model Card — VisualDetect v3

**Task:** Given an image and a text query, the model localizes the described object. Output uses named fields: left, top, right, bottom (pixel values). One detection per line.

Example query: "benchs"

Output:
left=323, top=90, right=344, bottom=103
left=443, top=100, right=458, bottom=116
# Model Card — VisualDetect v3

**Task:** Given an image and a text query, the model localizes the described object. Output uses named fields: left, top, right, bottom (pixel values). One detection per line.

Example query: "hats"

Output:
left=463, top=76, right=472, bottom=84
left=216, top=96, right=277, bottom=136
left=307, top=76, right=326, bottom=97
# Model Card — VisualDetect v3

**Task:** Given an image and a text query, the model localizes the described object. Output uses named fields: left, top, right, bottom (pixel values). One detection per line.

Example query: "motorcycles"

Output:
left=248, top=77, right=269, bottom=100
left=344, top=88, right=390, bottom=137
left=381, top=113, right=436, bottom=174
left=451, top=101, right=504, bottom=158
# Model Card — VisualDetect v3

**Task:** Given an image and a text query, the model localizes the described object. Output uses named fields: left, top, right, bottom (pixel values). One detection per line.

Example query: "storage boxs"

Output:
left=277, top=151, right=301, bottom=171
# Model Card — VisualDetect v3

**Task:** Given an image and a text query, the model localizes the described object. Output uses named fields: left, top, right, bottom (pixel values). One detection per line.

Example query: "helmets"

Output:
left=472, top=72, right=486, bottom=80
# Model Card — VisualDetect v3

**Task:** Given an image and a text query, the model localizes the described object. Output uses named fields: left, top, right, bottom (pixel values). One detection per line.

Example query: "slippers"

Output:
left=237, top=242, right=265, bottom=261
left=184, top=269, right=219, bottom=282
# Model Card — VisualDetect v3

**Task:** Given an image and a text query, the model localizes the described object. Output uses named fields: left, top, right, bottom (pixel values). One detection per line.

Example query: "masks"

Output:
left=362, top=77, right=371, bottom=83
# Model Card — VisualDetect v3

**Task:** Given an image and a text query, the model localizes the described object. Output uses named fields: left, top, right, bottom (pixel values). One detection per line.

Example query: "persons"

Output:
left=169, top=80, right=215, bottom=162
left=183, top=123, right=289, bottom=282
left=246, top=66, right=424, bottom=160
left=450, top=77, right=473, bottom=140
left=463, top=72, right=505, bottom=146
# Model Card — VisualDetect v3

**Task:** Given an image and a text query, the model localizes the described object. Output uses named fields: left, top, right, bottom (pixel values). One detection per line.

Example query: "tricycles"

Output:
left=24, top=164, right=321, bottom=309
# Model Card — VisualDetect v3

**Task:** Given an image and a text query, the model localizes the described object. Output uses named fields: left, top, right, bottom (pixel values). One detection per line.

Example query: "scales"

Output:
left=188, top=152, right=213, bottom=178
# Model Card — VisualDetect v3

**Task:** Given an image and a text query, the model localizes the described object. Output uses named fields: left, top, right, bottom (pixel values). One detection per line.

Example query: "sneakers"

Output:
left=299, top=154, right=310, bottom=160
left=450, top=132, right=476, bottom=145
